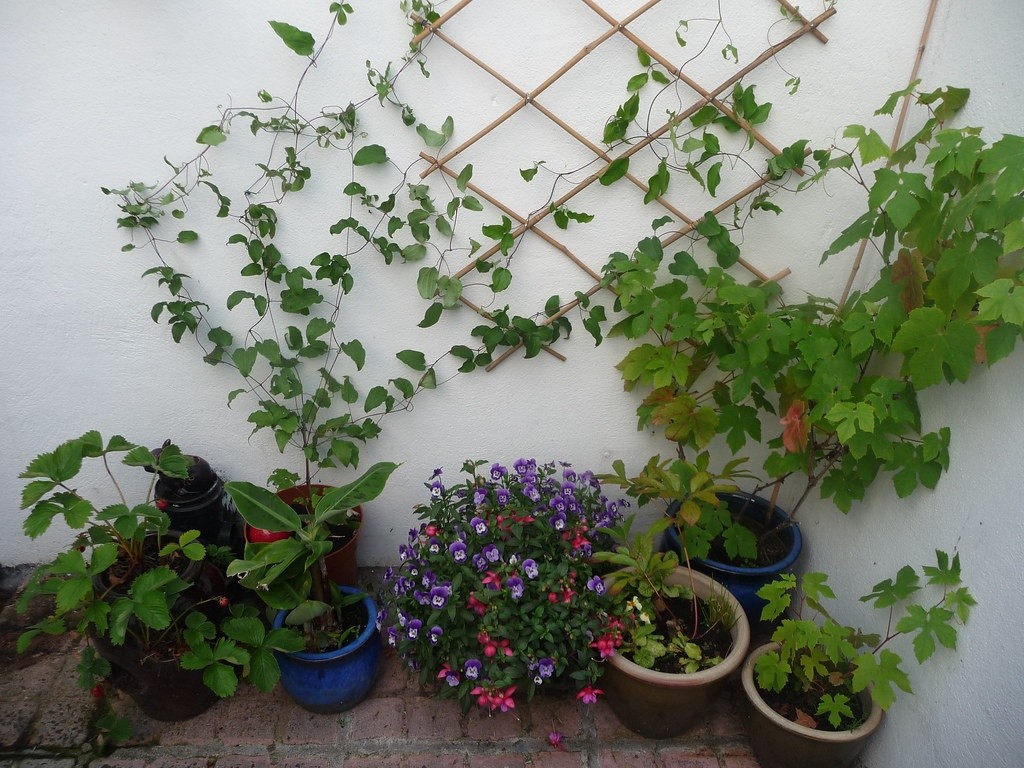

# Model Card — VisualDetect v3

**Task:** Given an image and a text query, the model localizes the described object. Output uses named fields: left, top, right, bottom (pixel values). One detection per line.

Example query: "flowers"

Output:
left=386, top=452, right=628, bottom=743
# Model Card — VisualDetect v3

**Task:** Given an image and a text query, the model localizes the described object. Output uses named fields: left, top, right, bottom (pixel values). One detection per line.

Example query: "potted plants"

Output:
left=581, top=355, right=754, bottom=737
left=19, top=424, right=235, bottom=718
left=605, top=84, right=1024, bottom=623
left=105, top=0, right=599, bottom=717
left=247, top=481, right=367, bottom=586
left=737, top=549, right=973, bottom=768
left=13, top=552, right=254, bottom=723
